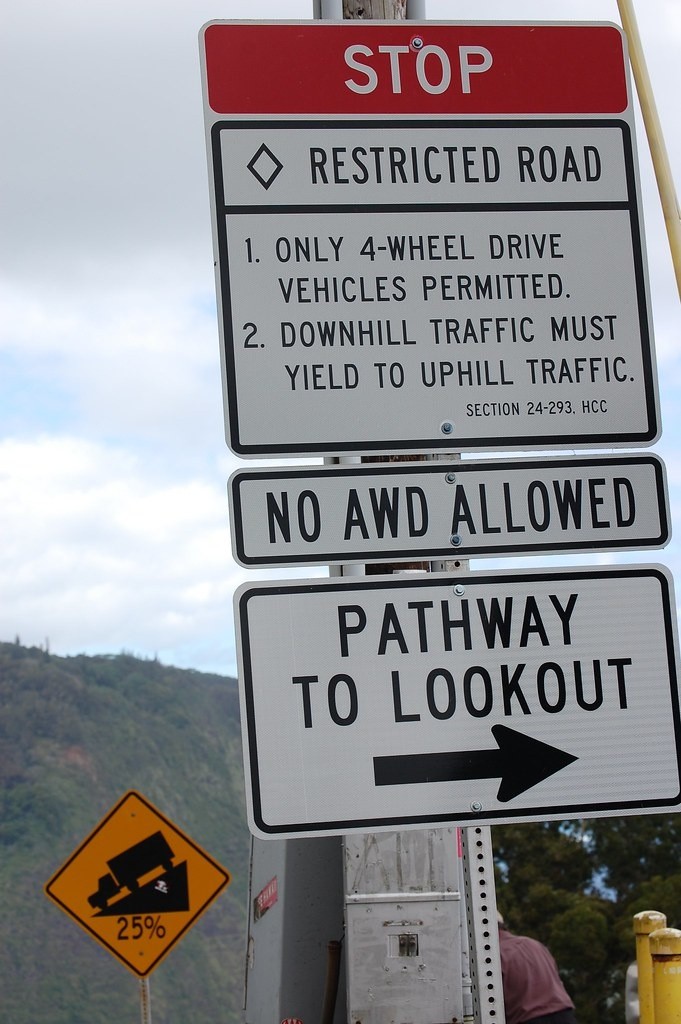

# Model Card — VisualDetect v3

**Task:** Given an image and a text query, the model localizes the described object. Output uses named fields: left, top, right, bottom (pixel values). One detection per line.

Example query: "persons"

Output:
left=496, top=909, right=577, bottom=1024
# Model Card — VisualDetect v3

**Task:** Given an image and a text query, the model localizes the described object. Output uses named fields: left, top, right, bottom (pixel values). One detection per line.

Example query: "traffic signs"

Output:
left=235, top=562, right=681, bottom=845
